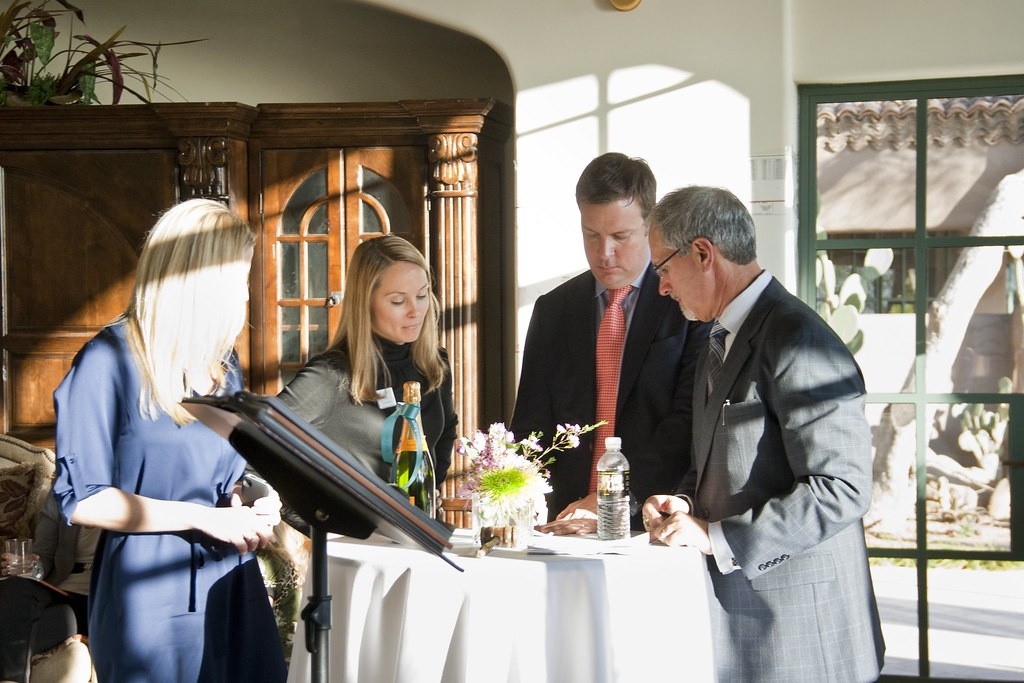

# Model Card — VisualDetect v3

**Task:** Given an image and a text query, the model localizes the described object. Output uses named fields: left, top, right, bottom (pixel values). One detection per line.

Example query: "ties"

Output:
left=589, top=285, right=631, bottom=494
left=705, top=321, right=730, bottom=397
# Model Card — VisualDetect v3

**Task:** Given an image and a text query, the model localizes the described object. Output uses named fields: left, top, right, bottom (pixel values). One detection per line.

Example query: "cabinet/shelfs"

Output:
left=1, top=98, right=514, bottom=452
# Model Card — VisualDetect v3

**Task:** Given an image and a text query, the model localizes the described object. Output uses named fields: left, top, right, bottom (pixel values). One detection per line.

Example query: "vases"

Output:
left=470, top=502, right=533, bottom=550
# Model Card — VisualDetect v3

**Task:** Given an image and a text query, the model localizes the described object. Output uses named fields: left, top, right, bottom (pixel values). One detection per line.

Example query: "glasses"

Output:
left=652, top=236, right=713, bottom=278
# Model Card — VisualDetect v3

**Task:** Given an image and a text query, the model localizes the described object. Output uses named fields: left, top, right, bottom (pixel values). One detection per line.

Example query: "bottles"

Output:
left=597, top=438, right=632, bottom=539
left=390, top=381, right=436, bottom=521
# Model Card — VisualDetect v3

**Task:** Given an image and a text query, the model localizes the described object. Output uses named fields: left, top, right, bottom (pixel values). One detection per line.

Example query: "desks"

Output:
left=286, top=529, right=716, bottom=683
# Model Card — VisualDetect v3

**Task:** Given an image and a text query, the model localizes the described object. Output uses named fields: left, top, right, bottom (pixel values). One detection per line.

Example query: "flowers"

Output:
left=457, top=418, right=609, bottom=511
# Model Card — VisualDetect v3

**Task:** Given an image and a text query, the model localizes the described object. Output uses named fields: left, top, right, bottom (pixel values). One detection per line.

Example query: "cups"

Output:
left=6, top=539, right=33, bottom=575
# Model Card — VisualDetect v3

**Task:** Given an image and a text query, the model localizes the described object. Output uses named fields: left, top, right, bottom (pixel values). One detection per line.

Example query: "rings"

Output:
left=643, top=517, right=649, bottom=525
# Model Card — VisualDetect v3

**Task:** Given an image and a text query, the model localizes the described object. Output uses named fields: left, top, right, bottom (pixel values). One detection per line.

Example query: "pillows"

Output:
left=0, top=461, right=45, bottom=553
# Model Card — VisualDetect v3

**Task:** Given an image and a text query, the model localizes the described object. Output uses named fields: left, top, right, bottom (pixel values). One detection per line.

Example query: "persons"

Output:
left=505, top=152, right=709, bottom=537
left=235, top=234, right=459, bottom=540
left=642, top=185, right=886, bottom=683
left=51, top=198, right=288, bottom=683
left=1, top=488, right=102, bottom=683
